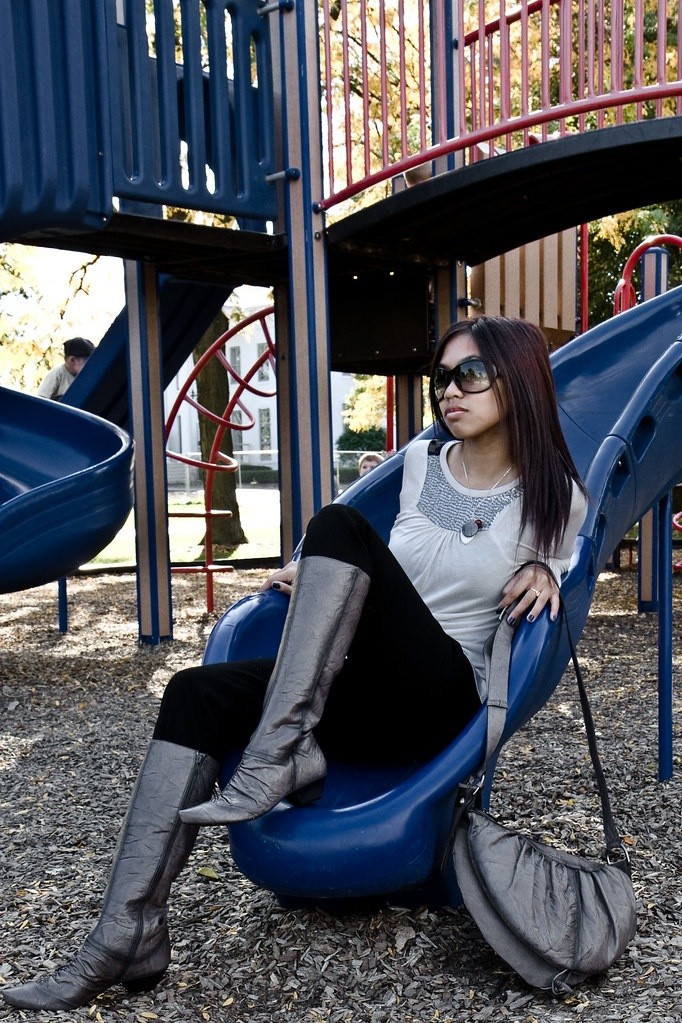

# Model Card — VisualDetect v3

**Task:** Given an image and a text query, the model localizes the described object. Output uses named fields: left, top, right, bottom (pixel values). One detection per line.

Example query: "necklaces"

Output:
left=457, top=442, right=516, bottom=546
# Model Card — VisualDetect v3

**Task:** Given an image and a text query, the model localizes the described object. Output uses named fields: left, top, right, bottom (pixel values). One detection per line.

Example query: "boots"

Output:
left=3, top=738, right=221, bottom=1011
left=175, top=554, right=372, bottom=826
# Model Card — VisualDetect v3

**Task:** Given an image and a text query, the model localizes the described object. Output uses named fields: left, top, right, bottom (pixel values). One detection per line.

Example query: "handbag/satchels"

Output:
left=454, top=811, right=635, bottom=992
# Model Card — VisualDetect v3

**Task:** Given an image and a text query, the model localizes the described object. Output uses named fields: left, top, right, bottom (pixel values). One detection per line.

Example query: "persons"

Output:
left=0, top=318, right=589, bottom=1010
left=356, top=452, right=387, bottom=478
left=36, top=335, right=98, bottom=402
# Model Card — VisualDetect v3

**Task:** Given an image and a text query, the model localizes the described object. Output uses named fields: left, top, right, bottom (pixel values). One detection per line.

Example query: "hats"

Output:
left=64, top=337, right=95, bottom=358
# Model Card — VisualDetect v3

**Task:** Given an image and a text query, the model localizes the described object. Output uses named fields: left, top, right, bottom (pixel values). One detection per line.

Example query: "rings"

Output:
left=530, top=587, right=542, bottom=596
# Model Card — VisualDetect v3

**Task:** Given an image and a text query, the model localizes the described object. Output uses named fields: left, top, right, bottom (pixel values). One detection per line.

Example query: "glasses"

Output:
left=433, top=359, right=501, bottom=403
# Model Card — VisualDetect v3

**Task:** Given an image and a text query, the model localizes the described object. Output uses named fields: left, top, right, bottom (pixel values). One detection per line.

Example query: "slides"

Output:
left=198, top=282, right=682, bottom=907
left=61, top=270, right=235, bottom=426
left=0, top=384, right=136, bottom=596
